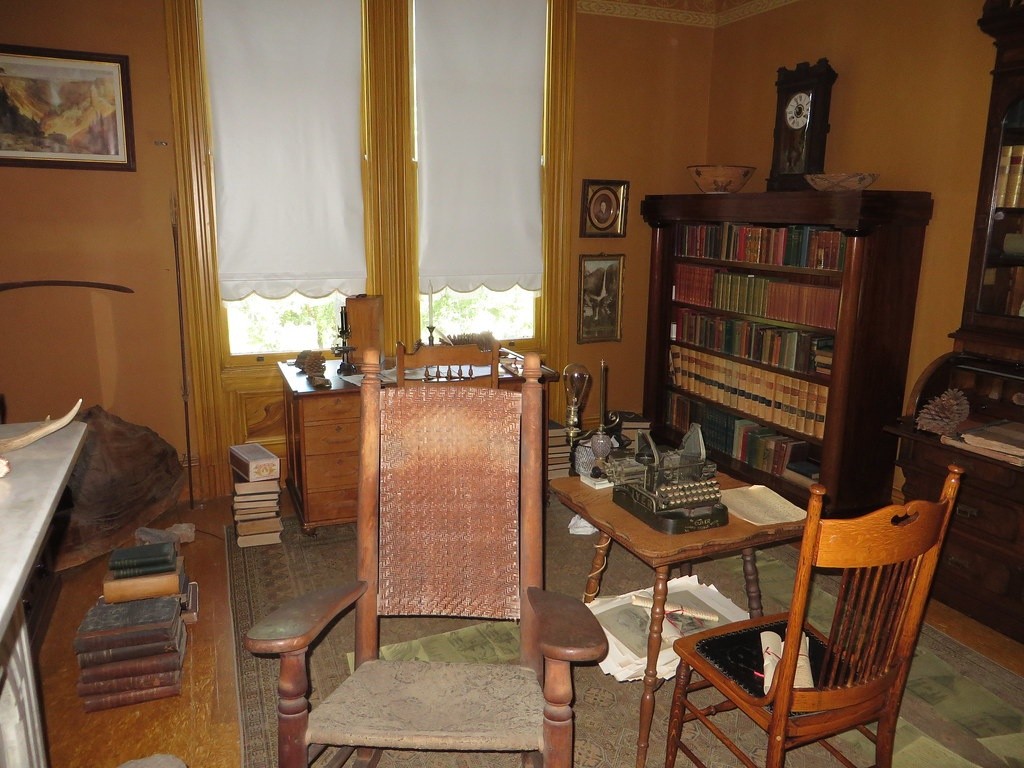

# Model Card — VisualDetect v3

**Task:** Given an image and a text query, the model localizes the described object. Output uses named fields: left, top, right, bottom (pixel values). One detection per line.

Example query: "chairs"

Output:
left=664, top=462, right=968, bottom=768
left=243, top=347, right=609, bottom=768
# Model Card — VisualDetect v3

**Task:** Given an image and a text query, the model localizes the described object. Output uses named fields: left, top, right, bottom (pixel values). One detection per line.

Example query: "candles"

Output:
left=428, top=279, right=433, bottom=327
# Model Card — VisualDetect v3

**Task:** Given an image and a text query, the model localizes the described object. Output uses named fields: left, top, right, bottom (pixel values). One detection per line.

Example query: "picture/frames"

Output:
left=579, top=178, right=631, bottom=239
left=577, top=254, right=626, bottom=345
left=0, top=43, right=137, bottom=173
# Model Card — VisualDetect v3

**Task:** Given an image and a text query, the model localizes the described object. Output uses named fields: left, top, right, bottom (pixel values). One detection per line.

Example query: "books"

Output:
left=606, top=410, right=651, bottom=454
left=580, top=475, right=614, bottom=490
left=547, top=421, right=571, bottom=480
left=228, top=443, right=284, bottom=548
left=72, top=542, right=199, bottom=712
left=980, top=145, right=1024, bottom=316
left=656, top=222, right=847, bottom=503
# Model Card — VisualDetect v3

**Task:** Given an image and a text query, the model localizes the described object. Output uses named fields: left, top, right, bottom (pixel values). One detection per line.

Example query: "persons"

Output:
left=594, top=199, right=609, bottom=223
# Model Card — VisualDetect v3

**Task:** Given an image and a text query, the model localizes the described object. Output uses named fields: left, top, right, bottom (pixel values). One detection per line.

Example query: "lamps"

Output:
left=562, top=356, right=610, bottom=480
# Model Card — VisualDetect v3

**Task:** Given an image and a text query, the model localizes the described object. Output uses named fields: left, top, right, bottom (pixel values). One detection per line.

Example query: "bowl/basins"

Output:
left=686, top=165, right=757, bottom=194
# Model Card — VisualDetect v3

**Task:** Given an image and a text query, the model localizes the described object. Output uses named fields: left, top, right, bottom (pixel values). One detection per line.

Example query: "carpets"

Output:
left=223, top=493, right=1024, bottom=768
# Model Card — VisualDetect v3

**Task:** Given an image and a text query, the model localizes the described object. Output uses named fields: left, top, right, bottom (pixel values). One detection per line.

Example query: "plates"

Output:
left=804, top=172, right=880, bottom=191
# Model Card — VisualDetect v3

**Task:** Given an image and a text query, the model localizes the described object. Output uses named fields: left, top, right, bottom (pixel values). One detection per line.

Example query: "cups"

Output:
left=591, top=435, right=611, bottom=458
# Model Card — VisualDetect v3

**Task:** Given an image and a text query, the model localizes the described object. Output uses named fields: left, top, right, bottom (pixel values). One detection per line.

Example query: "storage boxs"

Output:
left=229, top=443, right=281, bottom=483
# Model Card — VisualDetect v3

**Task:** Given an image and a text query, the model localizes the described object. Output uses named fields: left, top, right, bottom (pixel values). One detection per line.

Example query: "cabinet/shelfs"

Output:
left=883, top=0, right=1024, bottom=644
left=275, top=347, right=561, bottom=537
left=640, top=191, right=934, bottom=577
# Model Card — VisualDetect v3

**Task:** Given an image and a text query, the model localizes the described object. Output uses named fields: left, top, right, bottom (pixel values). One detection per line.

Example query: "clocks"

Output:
left=764, top=54, right=839, bottom=192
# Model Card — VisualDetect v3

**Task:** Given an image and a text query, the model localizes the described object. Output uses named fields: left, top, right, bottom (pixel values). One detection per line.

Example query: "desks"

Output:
left=0, top=420, right=90, bottom=768
left=549, top=475, right=806, bottom=768
